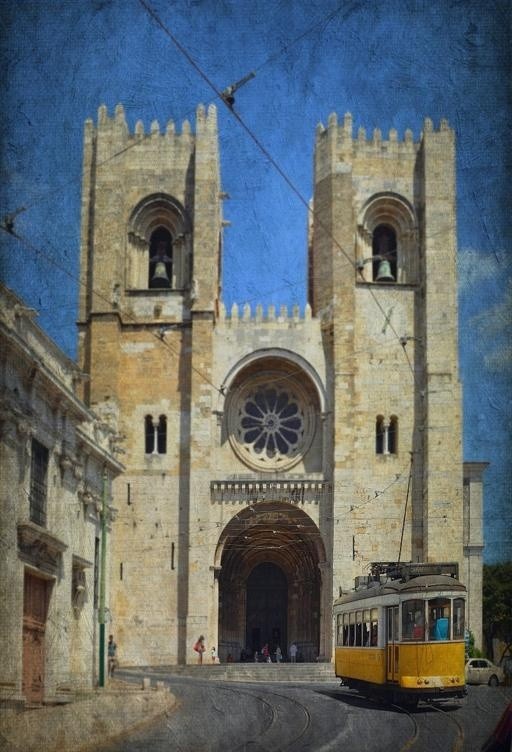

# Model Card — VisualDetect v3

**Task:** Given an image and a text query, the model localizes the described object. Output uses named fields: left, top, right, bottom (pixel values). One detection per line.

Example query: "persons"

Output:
left=197, top=634, right=204, bottom=665
left=343, top=605, right=457, bottom=646
left=108, top=634, right=117, bottom=677
left=218, top=642, right=305, bottom=663
left=211, top=647, right=216, bottom=664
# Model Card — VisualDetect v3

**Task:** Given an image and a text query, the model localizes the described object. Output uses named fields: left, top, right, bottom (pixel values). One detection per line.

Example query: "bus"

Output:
left=334, top=557, right=472, bottom=710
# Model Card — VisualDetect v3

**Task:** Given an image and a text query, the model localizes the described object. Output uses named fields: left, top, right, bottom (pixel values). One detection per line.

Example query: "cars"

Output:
left=466, top=658, right=506, bottom=686
